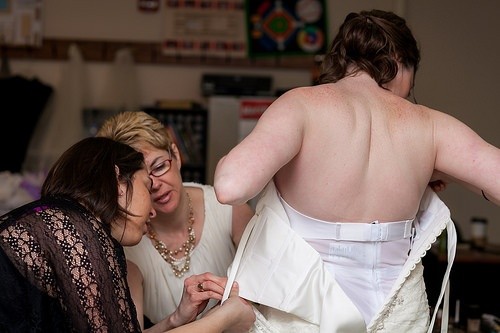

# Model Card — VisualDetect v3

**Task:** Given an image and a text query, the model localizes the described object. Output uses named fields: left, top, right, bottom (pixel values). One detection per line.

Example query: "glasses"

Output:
left=147, top=148, right=172, bottom=189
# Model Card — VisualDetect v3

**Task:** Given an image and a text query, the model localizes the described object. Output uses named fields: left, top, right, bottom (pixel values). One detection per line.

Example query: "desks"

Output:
left=422, top=242, right=500, bottom=333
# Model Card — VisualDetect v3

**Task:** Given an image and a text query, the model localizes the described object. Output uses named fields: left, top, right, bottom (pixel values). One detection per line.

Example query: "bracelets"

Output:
left=481, top=190, right=490, bottom=202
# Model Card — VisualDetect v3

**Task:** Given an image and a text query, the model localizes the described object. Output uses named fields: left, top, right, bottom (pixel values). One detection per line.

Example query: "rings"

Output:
left=198, top=283, right=204, bottom=291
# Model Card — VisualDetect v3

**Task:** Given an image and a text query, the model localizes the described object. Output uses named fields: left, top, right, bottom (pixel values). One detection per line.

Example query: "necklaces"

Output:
left=146, top=192, right=196, bottom=277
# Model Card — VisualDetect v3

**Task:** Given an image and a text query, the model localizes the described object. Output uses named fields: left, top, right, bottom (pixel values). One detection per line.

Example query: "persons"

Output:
left=96, top=112, right=256, bottom=333
left=0, top=136, right=256, bottom=332
left=213, top=10, right=500, bottom=333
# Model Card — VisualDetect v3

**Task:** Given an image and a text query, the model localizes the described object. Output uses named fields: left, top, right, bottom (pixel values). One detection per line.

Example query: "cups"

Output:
left=470, top=217, right=488, bottom=254
